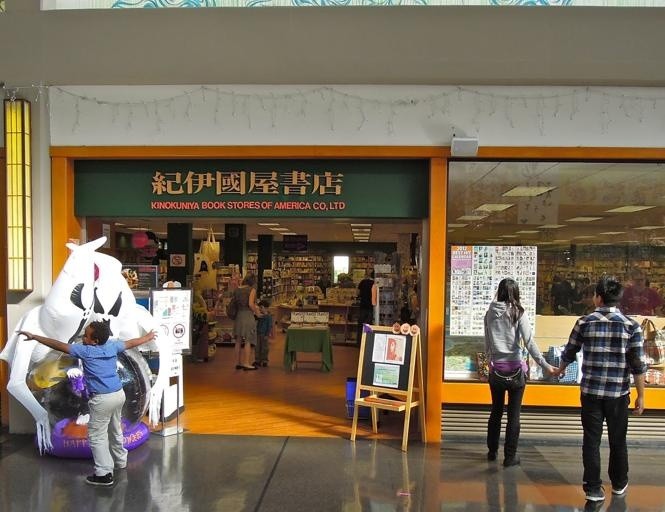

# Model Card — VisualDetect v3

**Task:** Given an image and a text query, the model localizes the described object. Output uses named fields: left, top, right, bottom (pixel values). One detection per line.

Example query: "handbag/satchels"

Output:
left=639, top=367, right=665, bottom=388
left=526, top=345, right=584, bottom=385
left=200, top=225, right=221, bottom=264
left=476, top=350, right=491, bottom=383
left=225, top=297, right=238, bottom=320
left=640, top=317, right=665, bottom=366
left=487, top=365, right=526, bottom=393
left=192, top=245, right=217, bottom=291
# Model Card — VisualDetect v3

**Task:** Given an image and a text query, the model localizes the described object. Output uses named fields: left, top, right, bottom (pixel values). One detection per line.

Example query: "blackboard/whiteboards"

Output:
left=357, top=326, right=417, bottom=396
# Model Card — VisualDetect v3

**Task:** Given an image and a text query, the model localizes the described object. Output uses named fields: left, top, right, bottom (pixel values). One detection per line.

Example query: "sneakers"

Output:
left=486, top=450, right=498, bottom=460
left=611, top=480, right=629, bottom=495
left=503, top=455, right=521, bottom=467
left=253, top=361, right=270, bottom=367
left=585, top=486, right=606, bottom=502
left=86, top=473, right=113, bottom=486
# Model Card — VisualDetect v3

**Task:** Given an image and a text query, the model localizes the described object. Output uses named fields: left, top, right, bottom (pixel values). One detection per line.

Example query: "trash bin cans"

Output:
left=345, top=377, right=371, bottom=420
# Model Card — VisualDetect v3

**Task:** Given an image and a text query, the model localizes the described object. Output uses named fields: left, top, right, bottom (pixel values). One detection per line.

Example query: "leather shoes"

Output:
left=242, top=364, right=258, bottom=371
left=235, top=364, right=244, bottom=369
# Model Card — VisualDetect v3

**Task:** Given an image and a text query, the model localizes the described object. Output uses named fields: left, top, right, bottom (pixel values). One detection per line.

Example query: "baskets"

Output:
left=344, top=376, right=372, bottom=419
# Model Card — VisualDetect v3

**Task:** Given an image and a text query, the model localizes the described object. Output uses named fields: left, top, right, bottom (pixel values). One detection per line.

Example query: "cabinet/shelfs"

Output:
left=192, top=252, right=398, bottom=375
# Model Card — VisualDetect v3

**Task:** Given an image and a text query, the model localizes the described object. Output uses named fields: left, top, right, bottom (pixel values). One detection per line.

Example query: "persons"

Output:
left=622, top=271, right=661, bottom=316
left=537, top=274, right=598, bottom=316
left=355, top=274, right=375, bottom=343
left=233, top=272, right=259, bottom=371
left=484, top=278, right=560, bottom=468
left=253, top=301, right=273, bottom=368
left=16, top=320, right=158, bottom=486
left=558, top=281, right=648, bottom=503
left=386, top=338, right=397, bottom=360
left=585, top=494, right=629, bottom=511
left=488, top=470, right=521, bottom=511
